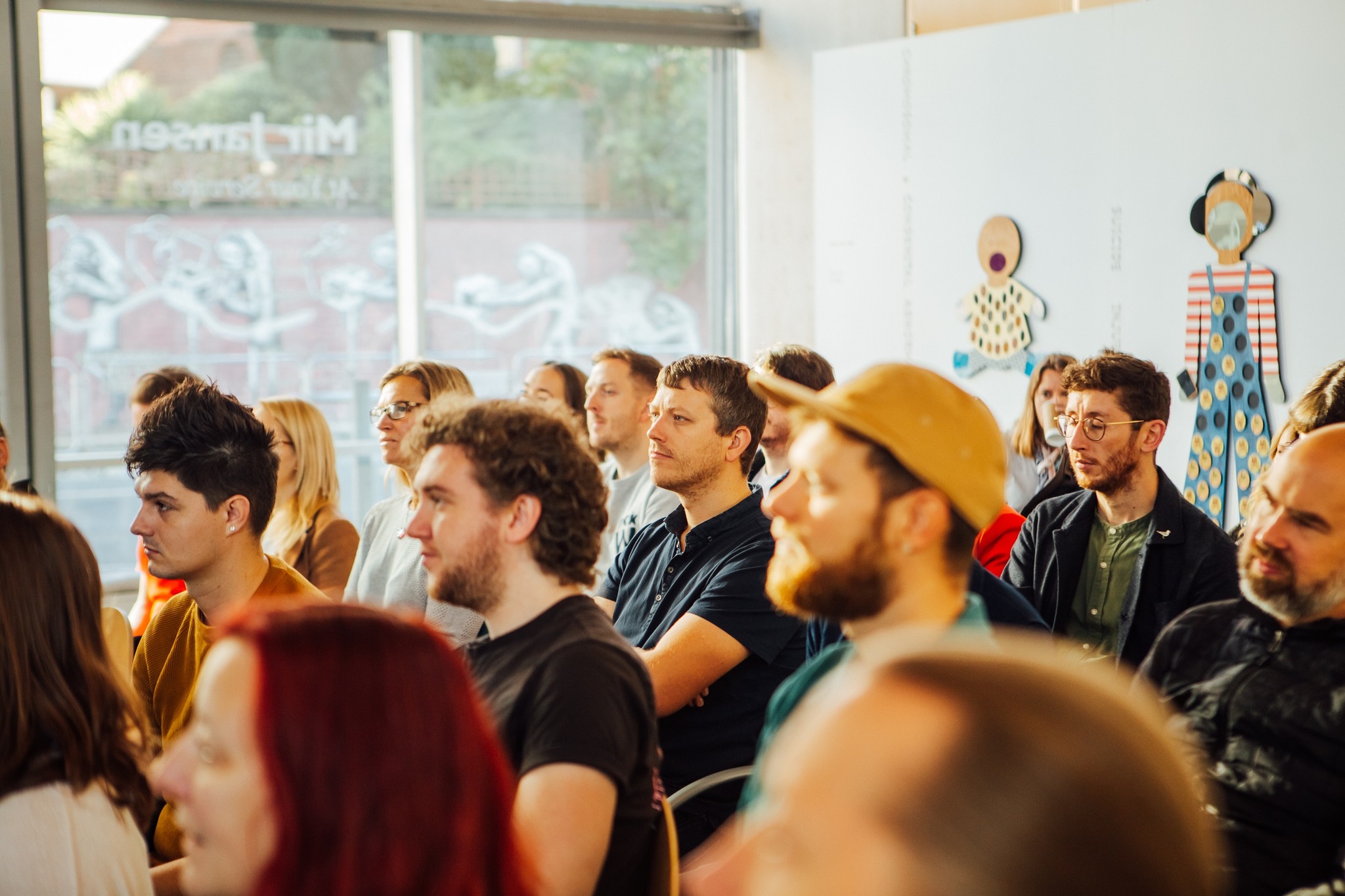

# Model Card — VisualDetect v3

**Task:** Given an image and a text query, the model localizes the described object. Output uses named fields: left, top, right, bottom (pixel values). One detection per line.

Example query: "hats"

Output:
left=746, top=363, right=1006, bottom=548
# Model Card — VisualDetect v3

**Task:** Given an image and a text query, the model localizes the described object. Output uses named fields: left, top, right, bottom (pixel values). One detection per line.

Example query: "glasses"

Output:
left=369, top=399, right=430, bottom=424
left=1053, top=414, right=1154, bottom=441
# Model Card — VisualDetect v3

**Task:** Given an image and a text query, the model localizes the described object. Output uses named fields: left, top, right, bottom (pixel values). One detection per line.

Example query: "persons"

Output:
left=0, top=350, right=1345, bottom=896
left=673, top=625, right=1233, bottom=895
